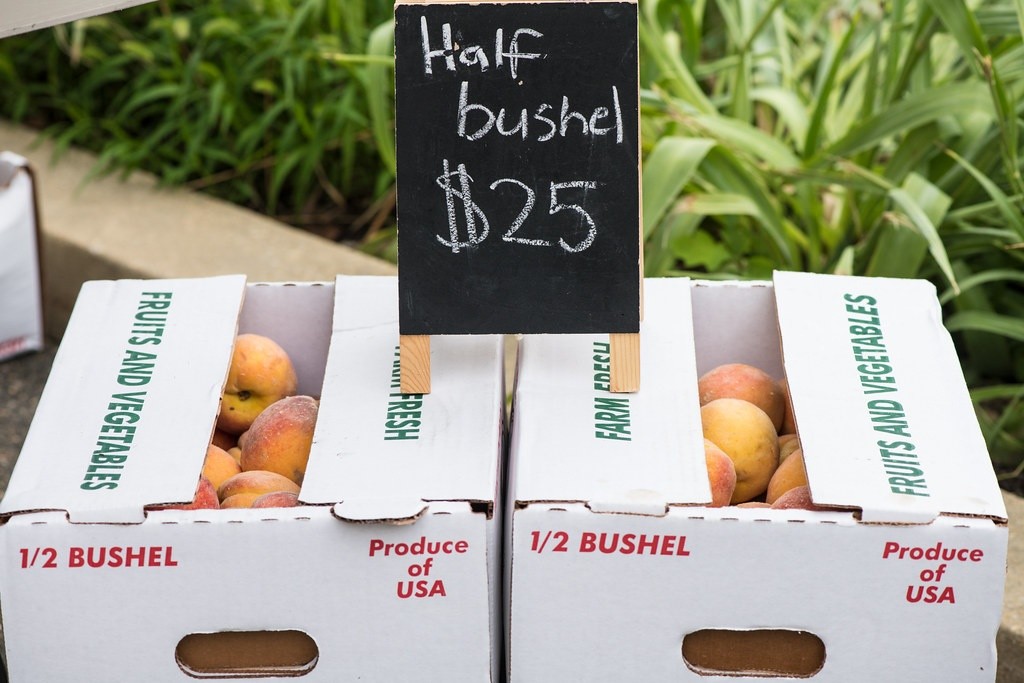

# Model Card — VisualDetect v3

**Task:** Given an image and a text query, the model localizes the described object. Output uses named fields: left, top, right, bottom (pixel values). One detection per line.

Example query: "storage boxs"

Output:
left=502, top=269, right=1005, bottom=682
left=0, top=274, right=503, bottom=682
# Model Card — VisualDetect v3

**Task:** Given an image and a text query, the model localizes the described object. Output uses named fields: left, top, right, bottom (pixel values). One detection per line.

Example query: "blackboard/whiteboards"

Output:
left=393, top=0, right=644, bottom=339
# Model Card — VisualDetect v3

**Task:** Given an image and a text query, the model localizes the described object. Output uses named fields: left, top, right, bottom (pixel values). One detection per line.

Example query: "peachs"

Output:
left=142, top=332, right=319, bottom=511
left=700, top=363, right=840, bottom=511
